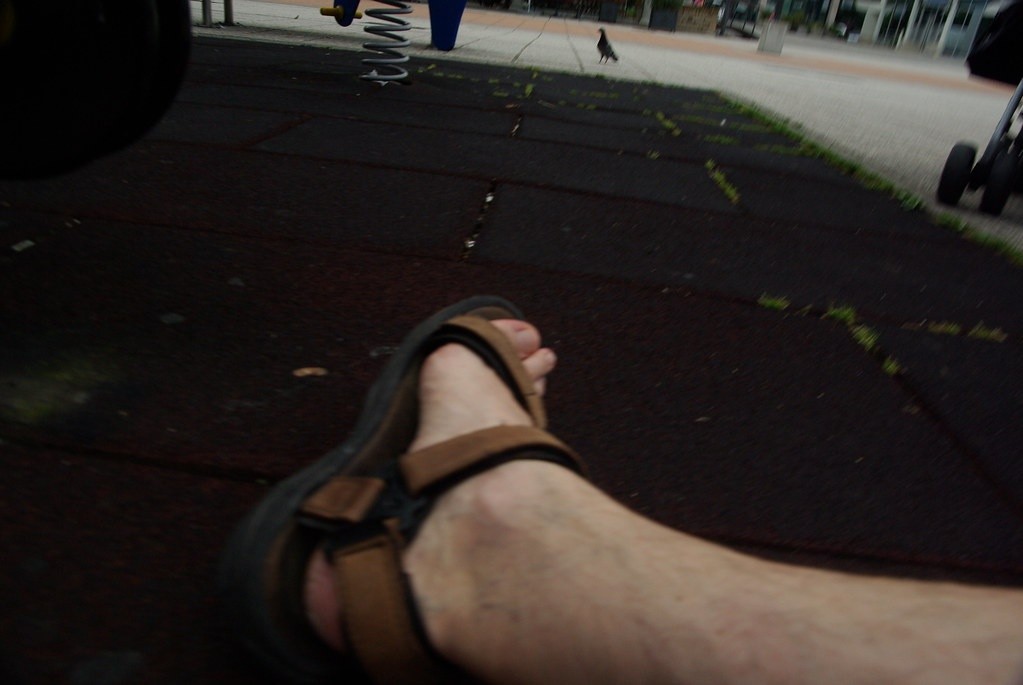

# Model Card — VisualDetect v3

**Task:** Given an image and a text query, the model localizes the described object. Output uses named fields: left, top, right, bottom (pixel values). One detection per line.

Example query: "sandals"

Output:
left=242, top=296, right=587, bottom=685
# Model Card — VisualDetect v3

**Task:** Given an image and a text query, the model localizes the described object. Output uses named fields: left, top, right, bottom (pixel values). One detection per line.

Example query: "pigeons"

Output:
left=597, top=27, right=619, bottom=63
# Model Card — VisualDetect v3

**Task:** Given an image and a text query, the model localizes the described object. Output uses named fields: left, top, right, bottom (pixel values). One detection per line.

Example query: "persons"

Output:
left=221, top=294, right=1023, bottom=685
